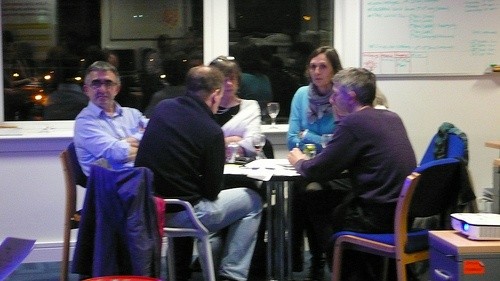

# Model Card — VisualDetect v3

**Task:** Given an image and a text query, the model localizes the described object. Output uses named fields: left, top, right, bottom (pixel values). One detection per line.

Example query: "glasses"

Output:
left=216, top=56, right=237, bottom=62
left=87, top=80, right=118, bottom=88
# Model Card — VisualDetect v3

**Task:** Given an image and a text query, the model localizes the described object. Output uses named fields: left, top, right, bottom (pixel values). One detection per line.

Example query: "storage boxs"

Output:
left=426, top=230, right=499, bottom=279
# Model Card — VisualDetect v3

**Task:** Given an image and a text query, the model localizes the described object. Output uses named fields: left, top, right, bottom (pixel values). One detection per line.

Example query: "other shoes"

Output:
left=190, top=258, right=203, bottom=272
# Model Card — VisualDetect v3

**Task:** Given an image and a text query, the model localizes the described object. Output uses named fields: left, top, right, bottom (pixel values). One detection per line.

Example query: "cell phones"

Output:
left=234, top=156, right=254, bottom=164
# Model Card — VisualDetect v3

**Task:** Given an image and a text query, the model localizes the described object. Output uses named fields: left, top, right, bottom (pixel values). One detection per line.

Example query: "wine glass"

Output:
left=252, top=133, right=265, bottom=161
left=266, top=102, right=280, bottom=126
left=321, top=133, right=334, bottom=149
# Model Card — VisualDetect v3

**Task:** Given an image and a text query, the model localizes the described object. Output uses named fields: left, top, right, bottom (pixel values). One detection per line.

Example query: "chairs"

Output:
left=329, top=158, right=467, bottom=279
left=60, top=142, right=107, bottom=280
left=88, top=164, right=216, bottom=281
left=418, top=124, right=470, bottom=183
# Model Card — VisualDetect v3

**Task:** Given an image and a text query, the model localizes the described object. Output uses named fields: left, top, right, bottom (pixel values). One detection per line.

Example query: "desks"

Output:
left=222, top=159, right=314, bottom=280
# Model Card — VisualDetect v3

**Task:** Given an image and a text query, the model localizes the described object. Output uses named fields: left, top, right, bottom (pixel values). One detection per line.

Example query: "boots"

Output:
left=292, top=247, right=303, bottom=271
left=304, top=253, right=326, bottom=281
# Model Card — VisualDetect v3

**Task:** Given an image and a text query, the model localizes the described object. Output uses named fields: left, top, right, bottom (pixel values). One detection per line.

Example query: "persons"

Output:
left=288, top=67, right=418, bottom=281
left=203, top=55, right=264, bottom=162
left=228, top=16, right=331, bottom=117
left=133, top=66, right=264, bottom=281
left=1, top=26, right=203, bottom=119
left=73, top=60, right=148, bottom=181
left=287, top=45, right=343, bottom=160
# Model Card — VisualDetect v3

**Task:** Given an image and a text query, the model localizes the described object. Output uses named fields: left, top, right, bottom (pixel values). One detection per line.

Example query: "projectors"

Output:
left=450, top=212, right=500, bottom=240
